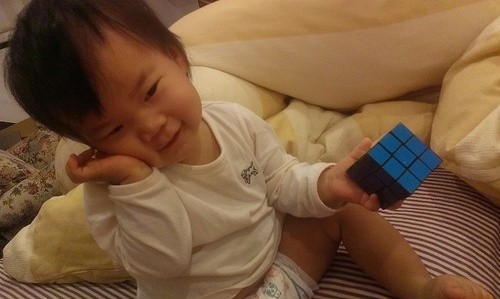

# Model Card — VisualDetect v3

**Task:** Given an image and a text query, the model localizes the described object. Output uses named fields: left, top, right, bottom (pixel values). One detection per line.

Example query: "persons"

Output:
left=5, top=1, right=498, bottom=299
